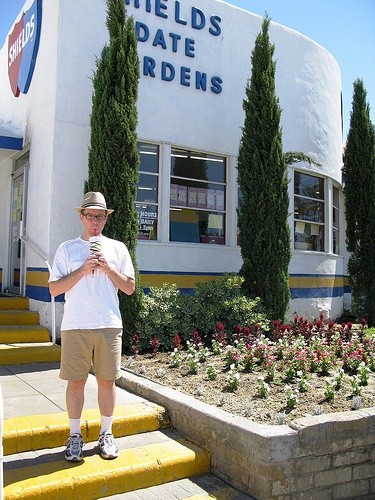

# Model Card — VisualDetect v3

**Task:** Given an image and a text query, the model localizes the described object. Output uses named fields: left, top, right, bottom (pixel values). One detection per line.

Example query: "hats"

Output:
left=75, top=191, right=114, bottom=214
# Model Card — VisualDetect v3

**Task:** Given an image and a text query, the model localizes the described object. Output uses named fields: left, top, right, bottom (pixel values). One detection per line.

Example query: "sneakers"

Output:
left=98, top=431, right=118, bottom=458
left=64, top=433, right=85, bottom=462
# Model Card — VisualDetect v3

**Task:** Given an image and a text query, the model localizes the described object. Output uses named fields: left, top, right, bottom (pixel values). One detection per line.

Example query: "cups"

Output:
left=89, top=236, right=101, bottom=261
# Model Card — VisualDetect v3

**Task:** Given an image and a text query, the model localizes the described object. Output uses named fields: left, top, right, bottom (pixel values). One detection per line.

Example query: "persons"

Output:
left=48, top=192, right=136, bottom=461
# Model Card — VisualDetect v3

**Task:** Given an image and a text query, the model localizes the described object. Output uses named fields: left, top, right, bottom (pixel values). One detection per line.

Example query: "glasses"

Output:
left=82, top=213, right=107, bottom=220
